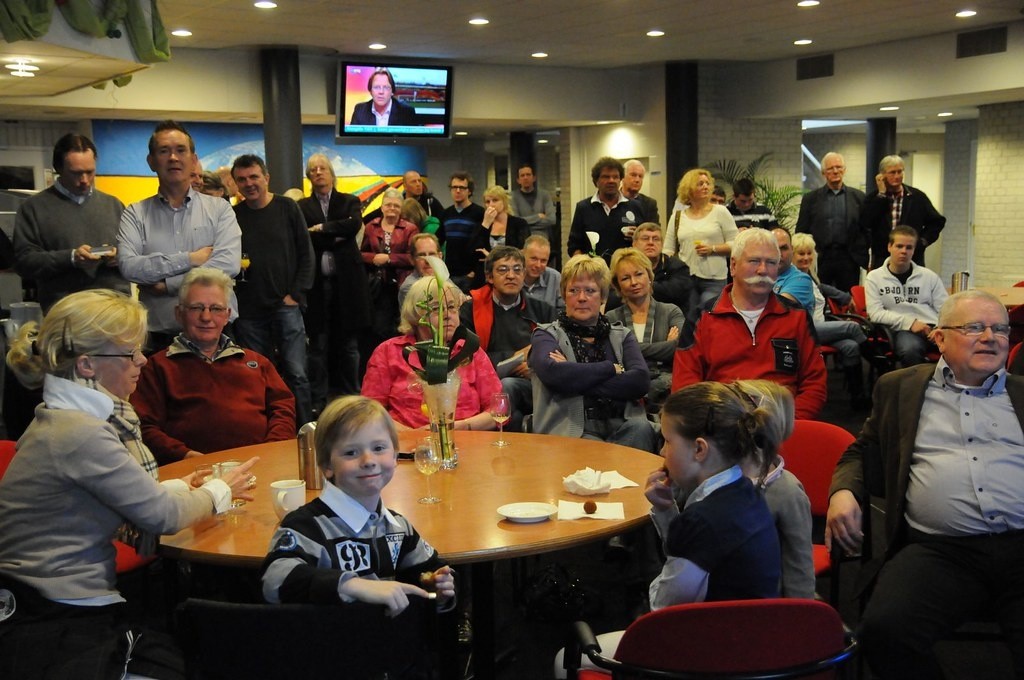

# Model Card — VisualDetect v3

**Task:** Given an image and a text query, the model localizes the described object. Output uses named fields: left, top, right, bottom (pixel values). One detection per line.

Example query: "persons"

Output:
left=824, top=290, right=1024, bottom=680
left=0, top=120, right=949, bottom=587
left=672, top=378, right=815, bottom=602
left=254, top=396, right=468, bottom=627
left=348, top=71, right=415, bottom=125
left=556, top=381, right=783, bottom=680
left=0, top=287, right=259, bottom=680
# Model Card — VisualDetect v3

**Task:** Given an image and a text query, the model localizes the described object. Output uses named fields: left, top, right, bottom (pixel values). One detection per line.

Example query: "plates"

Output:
left=496, top=502, right=557, bottom=522
left=203, top=474, right=256, bottom=486
left=9, top=302, right=42, bottom=330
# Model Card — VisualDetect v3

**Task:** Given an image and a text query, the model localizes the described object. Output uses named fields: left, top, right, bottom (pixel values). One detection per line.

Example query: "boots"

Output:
left=858, top=339, right=899, bottom=368
left=844, top=363, right=873, bottom=411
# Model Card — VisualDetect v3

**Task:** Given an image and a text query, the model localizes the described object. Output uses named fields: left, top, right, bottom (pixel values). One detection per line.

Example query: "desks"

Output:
left=150, top=421, right=671, bottom=680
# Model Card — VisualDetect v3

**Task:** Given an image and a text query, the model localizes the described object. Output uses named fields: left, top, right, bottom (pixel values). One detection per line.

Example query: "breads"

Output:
left=584, top=500, right=597, bottom=514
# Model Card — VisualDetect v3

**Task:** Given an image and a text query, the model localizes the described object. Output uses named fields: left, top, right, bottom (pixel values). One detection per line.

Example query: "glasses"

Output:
left=85, top=341, right=144, bottom=362
left=449, top=184, right=469, bottom=192
left=491, top=264, right=526, bottom=276
left=180, top=302, right=229, bottom=318
left=940, top=320, right=1011, bottom=338
left=419, top=299, right=459, bottom=314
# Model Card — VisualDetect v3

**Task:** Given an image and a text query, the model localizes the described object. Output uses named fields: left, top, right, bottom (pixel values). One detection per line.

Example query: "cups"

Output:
left=694, top=240, right=705, bottom=255
left=270, top=479, right=306, bottom=522
left=297, top=421, right=323, bottom=490
left=0, top=318, right=19, bottom=339
left=951, top=272, right=968, bottom=295
left=195, top=458, right=256, bottom=514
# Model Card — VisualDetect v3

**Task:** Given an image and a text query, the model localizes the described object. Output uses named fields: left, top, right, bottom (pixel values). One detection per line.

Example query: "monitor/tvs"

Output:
left=333, top=59, right=454, bottom=144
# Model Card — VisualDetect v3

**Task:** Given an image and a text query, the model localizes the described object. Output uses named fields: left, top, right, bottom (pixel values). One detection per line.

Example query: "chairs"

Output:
left=0, top=283, right=1023, bottom=680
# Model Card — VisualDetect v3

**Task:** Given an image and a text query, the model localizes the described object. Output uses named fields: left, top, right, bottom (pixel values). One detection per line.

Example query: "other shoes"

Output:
left=457, top=620, right=475, bottom=644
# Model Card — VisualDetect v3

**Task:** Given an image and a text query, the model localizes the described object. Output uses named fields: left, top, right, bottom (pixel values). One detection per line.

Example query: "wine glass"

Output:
left=239, top=253, right=251, bottom=282
left=414, top=435, right=442, bottom=505
left=491, top=393, right=511, bottom=447
